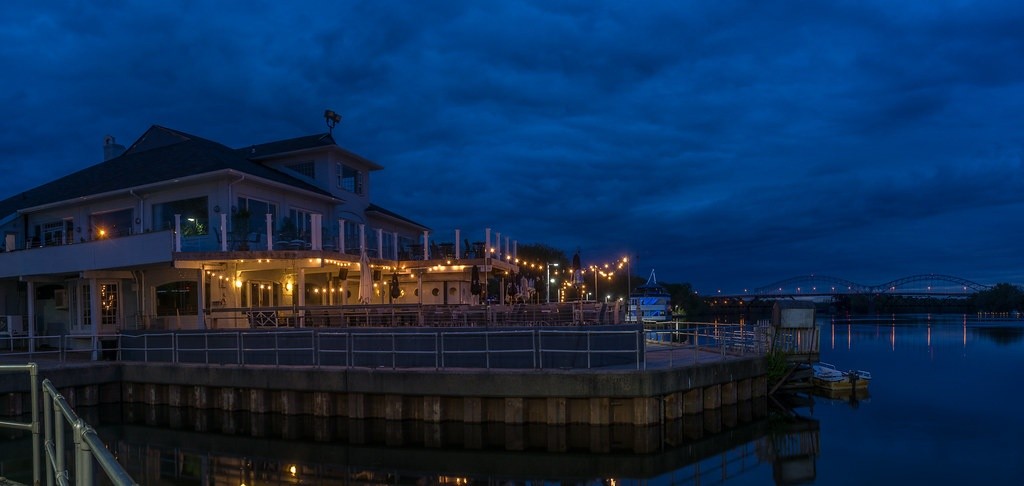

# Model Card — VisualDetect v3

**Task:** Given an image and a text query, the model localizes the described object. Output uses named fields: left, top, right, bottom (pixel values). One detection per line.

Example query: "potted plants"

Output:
left=238, top=223, right=250, bottom=251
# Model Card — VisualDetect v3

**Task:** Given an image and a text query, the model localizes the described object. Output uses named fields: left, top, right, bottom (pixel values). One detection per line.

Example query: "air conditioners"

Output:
left=54, top=289, right=69, bottom=310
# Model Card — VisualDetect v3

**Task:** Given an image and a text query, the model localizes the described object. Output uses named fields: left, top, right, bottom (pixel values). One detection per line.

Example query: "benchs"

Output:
left=248, top=302, right=625, bottom=326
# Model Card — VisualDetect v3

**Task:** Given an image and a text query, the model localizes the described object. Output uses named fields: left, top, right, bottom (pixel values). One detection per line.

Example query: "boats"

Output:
left=627, top=269, right=672, bottom=329
left=811, top=361, right=871, bottom=392
